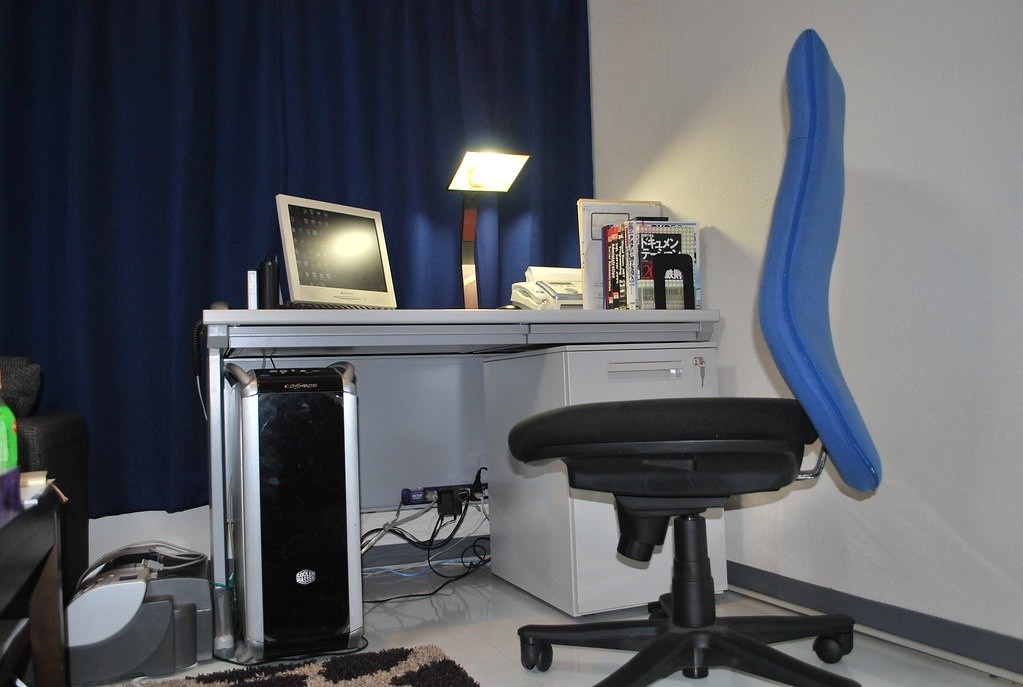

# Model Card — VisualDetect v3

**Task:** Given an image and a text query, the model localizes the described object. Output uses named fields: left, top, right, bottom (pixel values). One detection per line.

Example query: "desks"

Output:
left=199, top=307, right=722, bottom=658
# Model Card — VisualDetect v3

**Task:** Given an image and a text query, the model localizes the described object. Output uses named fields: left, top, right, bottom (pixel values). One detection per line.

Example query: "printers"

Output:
left=64, top=544, right=217, bottom=687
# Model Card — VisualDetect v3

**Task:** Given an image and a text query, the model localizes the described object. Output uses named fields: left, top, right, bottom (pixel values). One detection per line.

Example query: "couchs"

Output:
left=0, top=354, right=89, bottom=622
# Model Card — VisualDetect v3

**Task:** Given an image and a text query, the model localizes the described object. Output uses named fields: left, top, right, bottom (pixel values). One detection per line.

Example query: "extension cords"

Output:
left=402, top=483, right=489, bottom=505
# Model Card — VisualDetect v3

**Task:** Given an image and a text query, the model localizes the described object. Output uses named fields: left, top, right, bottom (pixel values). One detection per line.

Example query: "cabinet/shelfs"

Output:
left=483, top=342, right=728, bottom=619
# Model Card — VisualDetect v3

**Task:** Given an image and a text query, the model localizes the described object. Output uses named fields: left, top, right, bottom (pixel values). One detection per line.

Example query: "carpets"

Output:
left=118, top=646, right=480, bottom=687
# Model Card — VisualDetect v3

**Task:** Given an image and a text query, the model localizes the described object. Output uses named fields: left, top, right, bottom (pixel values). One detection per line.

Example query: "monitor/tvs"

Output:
left=276, top=194, right=398, bottom=310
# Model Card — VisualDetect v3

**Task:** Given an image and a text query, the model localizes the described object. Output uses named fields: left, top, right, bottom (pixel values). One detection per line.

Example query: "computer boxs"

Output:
left=223, top=362, right=364, bottom=659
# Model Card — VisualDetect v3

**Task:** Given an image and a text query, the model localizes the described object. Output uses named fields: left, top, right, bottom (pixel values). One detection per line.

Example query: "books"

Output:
left=19, top=470, right=48, bottom=487
left=510, top=197, right=700, bottom=309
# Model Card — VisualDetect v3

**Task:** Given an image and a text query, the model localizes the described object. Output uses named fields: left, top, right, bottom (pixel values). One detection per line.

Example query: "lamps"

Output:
left=447, top=144, right=531, bottom=310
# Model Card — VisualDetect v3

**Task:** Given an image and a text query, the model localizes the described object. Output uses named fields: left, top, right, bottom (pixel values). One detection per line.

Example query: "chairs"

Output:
left=507, top=28, right=882, bottom=687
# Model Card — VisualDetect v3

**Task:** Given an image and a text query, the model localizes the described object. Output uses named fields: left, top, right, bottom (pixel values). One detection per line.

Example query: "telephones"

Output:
left=509, top=280, right=583, bottom=310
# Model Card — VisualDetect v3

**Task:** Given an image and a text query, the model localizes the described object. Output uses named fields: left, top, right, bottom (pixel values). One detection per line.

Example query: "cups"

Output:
left=259, top=262, right=280, bottom=309
